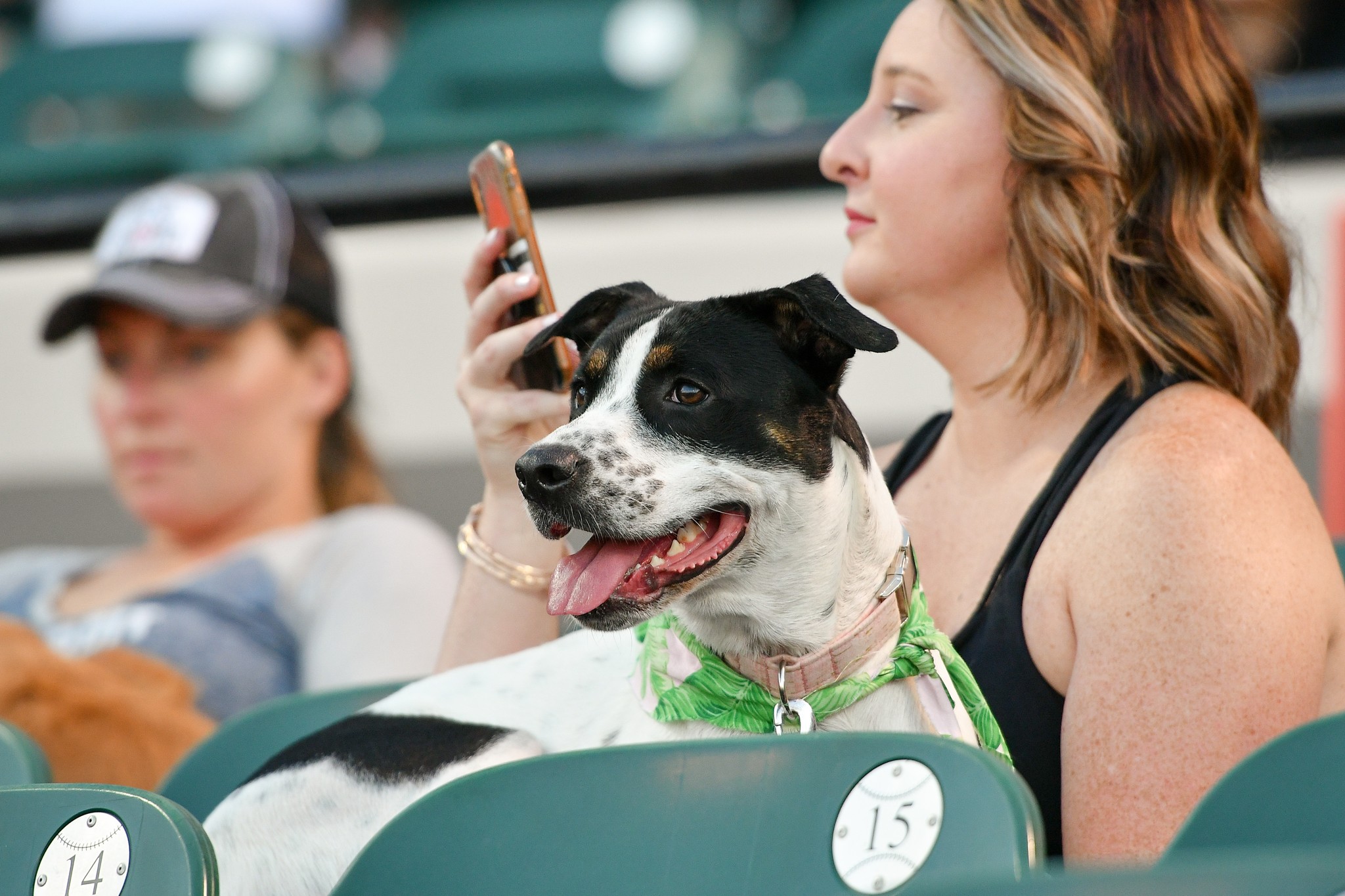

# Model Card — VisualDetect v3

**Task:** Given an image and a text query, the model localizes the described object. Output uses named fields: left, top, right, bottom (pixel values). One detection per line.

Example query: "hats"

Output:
left=41, top=169, right=339, bottom=349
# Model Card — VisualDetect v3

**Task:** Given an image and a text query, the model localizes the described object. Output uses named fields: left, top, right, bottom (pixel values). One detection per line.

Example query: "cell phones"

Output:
left=467, top=140, right=575, bottom=390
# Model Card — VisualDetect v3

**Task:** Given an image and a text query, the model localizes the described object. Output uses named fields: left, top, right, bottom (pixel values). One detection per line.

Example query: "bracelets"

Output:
left=457, top=499, right=575, bottom=594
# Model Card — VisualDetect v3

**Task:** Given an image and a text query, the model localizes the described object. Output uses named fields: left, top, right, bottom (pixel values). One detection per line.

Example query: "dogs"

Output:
left=200, top=275, right=973, bottom=896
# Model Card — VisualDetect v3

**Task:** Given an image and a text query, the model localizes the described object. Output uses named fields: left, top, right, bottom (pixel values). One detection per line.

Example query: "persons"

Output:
left=439, top=0, right=1345, bottom=869
left=0, top=170, right=461, bottom=725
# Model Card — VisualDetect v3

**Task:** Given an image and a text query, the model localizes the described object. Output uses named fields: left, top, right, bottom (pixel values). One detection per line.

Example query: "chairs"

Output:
left=334, top=739, right=1055, bottom=896
left=1, top=781, right=222, bottom=896
